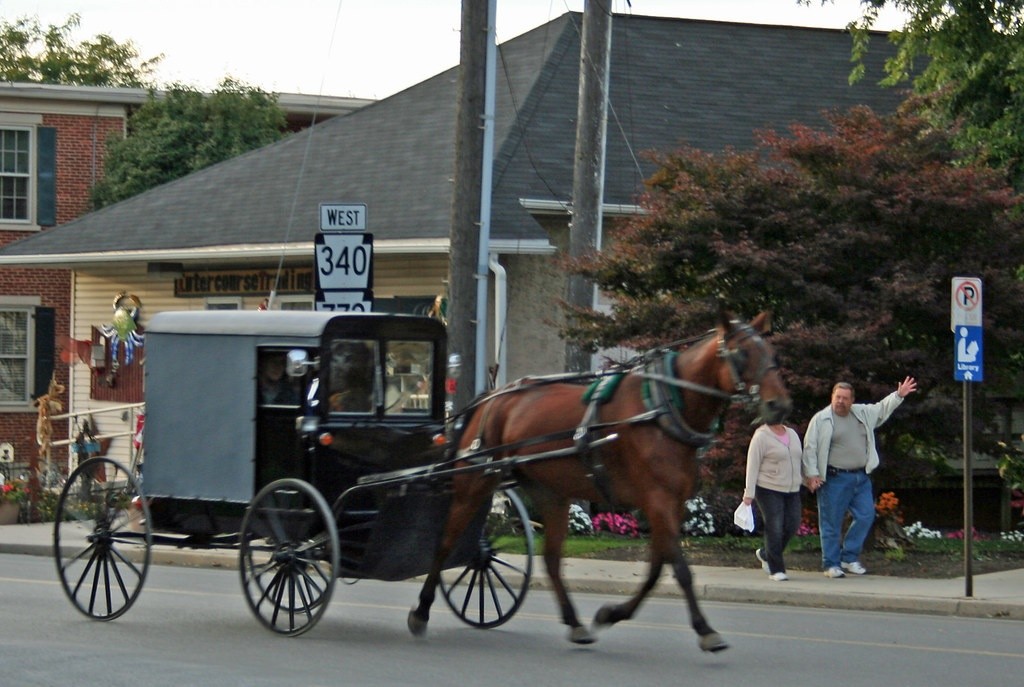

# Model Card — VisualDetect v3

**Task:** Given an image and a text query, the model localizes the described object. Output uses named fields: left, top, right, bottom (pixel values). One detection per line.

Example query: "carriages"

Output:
left=53, top=308, right=794, bottom=656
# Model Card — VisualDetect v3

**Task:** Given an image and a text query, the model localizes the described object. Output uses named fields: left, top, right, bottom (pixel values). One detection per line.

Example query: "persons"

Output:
left=803, top=376, right=916, bottom=578
left=743, top=424, right=815, bottom=580
left=262, top=354, right=298, bottom=404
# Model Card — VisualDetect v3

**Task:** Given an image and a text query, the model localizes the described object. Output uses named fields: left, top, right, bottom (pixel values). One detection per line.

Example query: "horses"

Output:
left=408, top=305, right=794, bottom=653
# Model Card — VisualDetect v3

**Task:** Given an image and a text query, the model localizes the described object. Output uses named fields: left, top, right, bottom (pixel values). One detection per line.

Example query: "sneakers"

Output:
left=841, top=561, right=866, bottom=574
left=769, top=572, right=788, bottom=581
left=824, top=566, right=844, bottom=577
left=756, top=549, right=770, bottom=573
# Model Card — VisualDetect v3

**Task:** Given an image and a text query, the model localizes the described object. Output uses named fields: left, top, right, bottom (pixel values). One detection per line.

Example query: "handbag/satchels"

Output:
left=734, top=501, right=754, bottom=533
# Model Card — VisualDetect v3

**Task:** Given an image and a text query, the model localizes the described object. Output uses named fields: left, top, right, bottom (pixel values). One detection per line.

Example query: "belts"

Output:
left=828, top=464, right=865, bottom=472
